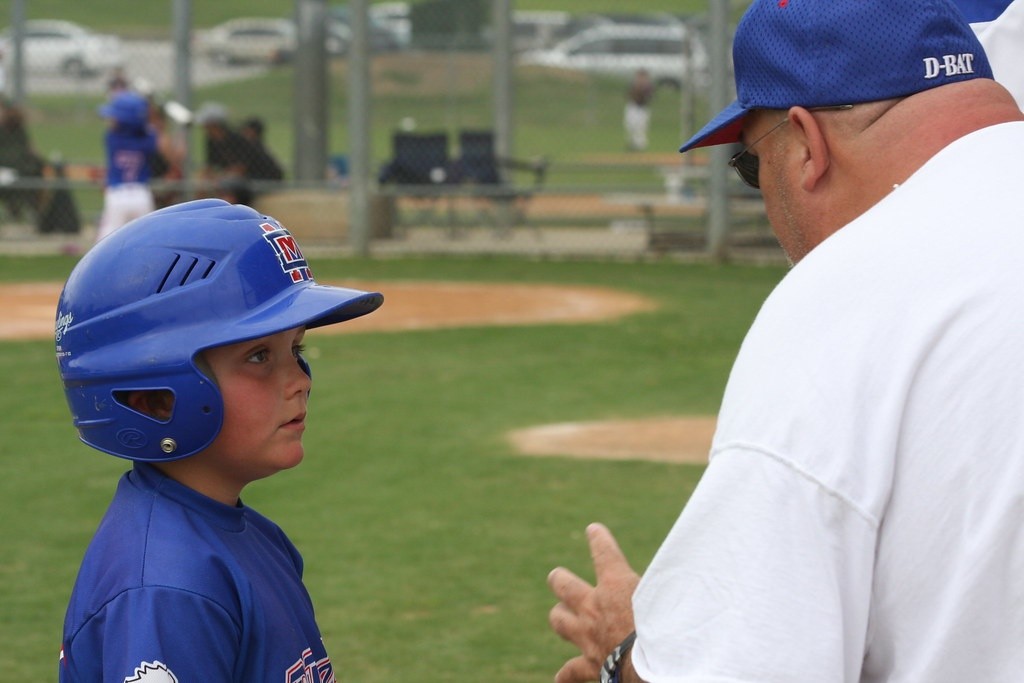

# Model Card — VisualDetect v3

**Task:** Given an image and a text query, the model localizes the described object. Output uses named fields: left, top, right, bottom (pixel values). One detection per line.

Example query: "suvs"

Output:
left=516, top=11, right=711, bottom=91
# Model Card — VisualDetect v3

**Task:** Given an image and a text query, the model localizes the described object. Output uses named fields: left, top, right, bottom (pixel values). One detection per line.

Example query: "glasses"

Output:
left=728, top=105, right=852, bottom=189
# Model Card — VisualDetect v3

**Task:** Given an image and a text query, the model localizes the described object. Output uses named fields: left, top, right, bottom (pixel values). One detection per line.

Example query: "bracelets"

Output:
left=600, top=629, right=636, bottom=683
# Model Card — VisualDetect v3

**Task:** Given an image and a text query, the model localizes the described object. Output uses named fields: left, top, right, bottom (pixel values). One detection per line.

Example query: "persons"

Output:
left=54, top=198, right=341, bottom=683
left=0, top=62, right=283, bottom=246
left=622, top=68, right=655, bottom=150
left=549, top=0, right=1024, bottom=683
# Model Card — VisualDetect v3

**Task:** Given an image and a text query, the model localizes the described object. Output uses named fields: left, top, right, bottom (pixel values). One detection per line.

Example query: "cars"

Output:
left=188, top=2, right=403, bottom=67
left=0, top=19, right=125, bottom=84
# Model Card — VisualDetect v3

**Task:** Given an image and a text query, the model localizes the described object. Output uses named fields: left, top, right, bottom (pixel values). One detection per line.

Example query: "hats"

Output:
left=679, top=1, right=995, bottom=153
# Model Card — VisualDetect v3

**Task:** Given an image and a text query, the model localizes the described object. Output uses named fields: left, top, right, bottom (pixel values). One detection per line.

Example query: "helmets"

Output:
left=99, top=93, right=146, bottom=124
left=57, top=198, right=384, bottom=462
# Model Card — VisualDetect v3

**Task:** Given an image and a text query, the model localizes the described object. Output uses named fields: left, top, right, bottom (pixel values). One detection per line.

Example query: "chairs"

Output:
left=382, top=127, right=547, bottom=242
